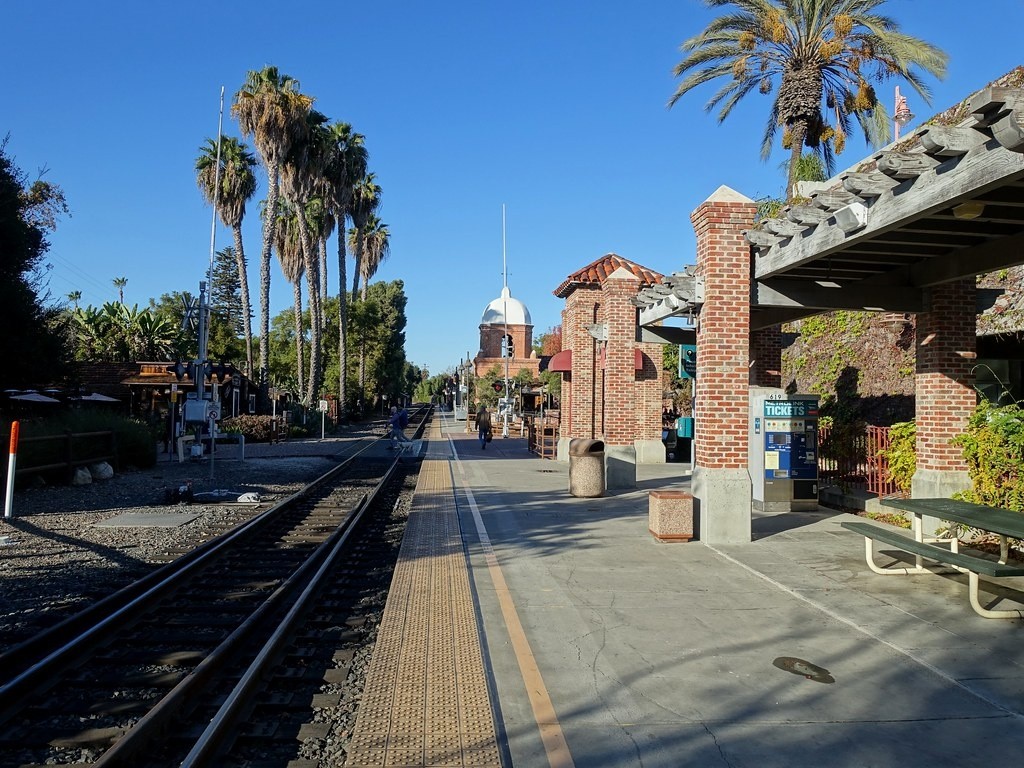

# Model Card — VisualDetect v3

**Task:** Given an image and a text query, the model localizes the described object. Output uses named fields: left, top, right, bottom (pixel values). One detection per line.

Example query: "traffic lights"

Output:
left=492, top=380, right=502, bottom=393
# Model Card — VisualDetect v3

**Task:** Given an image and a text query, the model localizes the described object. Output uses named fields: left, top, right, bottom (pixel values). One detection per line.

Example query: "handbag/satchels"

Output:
left=486, top=428, right=492, bottom=443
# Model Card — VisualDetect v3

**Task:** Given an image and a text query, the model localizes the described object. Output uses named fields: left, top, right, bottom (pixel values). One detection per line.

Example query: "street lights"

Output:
left=464, top=351, right=472, bottom=433
left=459, top=358, right=465, bottom=412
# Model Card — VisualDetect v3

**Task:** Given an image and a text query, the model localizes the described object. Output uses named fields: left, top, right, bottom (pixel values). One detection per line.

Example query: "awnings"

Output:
left=548, top=350, right=571, bottom=372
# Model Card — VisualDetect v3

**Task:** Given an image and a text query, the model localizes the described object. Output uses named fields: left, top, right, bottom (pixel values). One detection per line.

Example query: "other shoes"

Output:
left=482, top=444, right=485, bottom=450
left=384, top=446, right=393, bottom=450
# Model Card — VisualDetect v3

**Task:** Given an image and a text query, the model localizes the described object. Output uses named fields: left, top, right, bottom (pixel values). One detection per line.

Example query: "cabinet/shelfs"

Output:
left=528, top=424, right=555, bottom=459
left=467, top=414, right=480, bottom=434
left=491, top=421, right=527, bottom=438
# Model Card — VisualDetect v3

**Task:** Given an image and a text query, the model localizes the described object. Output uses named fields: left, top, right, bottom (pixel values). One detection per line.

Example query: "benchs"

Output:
left=840, top=522, right=1024, bottom=617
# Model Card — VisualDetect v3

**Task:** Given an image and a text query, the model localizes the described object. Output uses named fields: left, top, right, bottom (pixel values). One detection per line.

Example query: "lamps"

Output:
left=687, top=308, right=694, bottom=327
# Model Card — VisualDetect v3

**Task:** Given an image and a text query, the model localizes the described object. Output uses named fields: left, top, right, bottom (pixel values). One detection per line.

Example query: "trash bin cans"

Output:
left=648, top=490, right=694, bottom=543
left=569, top=438, right=605, bottom=498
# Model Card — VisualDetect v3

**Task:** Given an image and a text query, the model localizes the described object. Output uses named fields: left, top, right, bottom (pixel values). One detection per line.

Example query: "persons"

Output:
left=662, top=409, right=674, bottom=426
left=203, top=440, right=217, bottom=455
left=475, top=405, right=491, bottom=450
left=536, top=401, right=559, bottom=412
left=386, top=404, right=412, bottom=450
left=161, top=402, right=179, bottom=454
left=515, top=403, right=532, bottom=412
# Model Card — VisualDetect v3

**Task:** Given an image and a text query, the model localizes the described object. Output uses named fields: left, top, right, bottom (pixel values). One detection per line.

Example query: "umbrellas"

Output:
left=4, top=388, right=122, bottom=402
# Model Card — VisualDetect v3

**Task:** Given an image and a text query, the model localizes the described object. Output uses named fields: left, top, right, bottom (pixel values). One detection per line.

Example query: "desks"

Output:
left=880, top=498, right=1024, bottom=574
